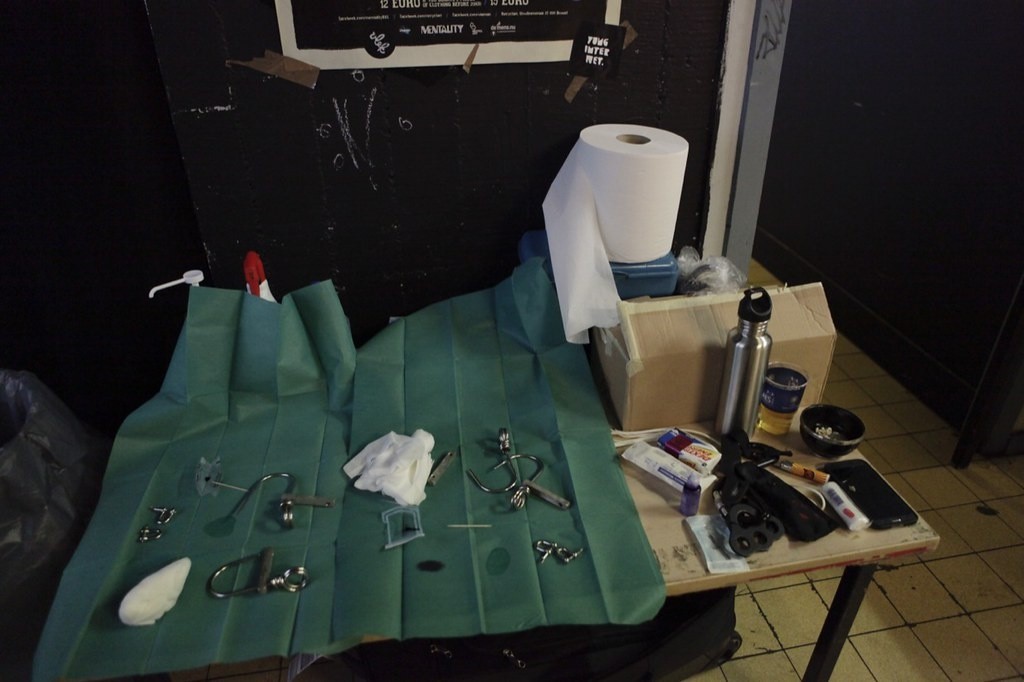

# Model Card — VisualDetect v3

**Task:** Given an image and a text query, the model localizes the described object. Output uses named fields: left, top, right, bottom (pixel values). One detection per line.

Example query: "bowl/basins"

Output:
left=800, top=404, right=866, bottom=457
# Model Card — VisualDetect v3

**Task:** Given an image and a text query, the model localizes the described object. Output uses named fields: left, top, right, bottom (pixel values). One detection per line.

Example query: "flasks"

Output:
left=715, top=287, right=773, bottom=443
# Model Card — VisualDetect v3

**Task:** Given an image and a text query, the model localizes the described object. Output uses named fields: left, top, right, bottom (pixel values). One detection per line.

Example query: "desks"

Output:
left=610, top=402, right=938, bottom=681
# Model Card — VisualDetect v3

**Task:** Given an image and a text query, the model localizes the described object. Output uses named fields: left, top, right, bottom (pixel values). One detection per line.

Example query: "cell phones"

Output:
left=823, top=459, right=919, bottom=530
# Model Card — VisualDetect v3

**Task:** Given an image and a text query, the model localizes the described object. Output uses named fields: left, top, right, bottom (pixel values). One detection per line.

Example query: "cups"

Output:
left=758, top=361, right=809, bottom=436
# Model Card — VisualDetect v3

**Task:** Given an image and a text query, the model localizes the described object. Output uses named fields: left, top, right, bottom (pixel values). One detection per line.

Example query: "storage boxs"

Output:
left=595, top=277, right=839, bottom=432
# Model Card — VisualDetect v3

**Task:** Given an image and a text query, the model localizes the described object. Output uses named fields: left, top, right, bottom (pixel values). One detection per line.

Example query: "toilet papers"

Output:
left=542, top=122, right=691, bottom=344
